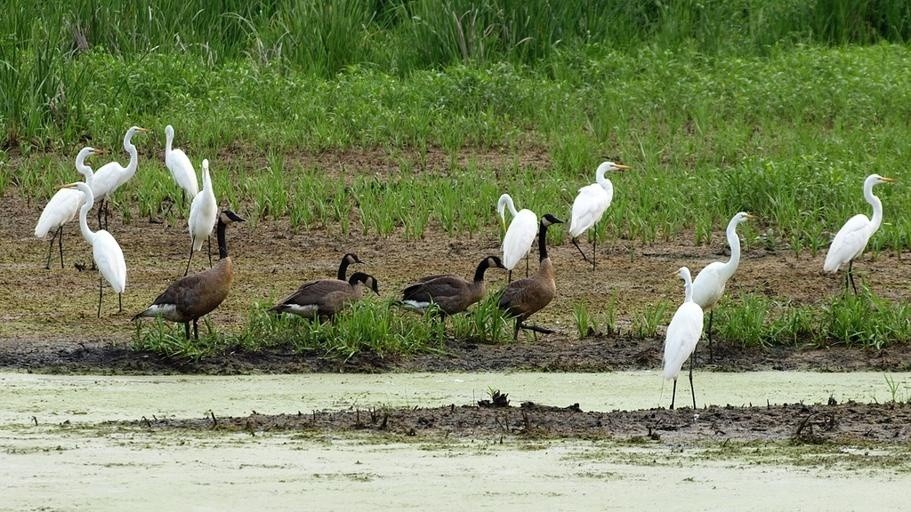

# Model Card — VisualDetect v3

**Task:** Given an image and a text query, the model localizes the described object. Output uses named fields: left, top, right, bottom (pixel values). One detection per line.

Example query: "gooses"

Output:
left=265, top=252, right=380, bottom=324
left=129, top=209, right=246, bottom=341
left=388, top=256, right=506, bottom=337
left=480, top=214, right=564, bottom=341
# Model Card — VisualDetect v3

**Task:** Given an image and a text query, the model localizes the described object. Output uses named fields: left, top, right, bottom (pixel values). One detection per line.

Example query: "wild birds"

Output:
left=35, top=123, right=218, bottom=318
left=822, top=173, right=898, bottom=299
left=494, top=192, right=540, bottom=283
left=661, top=212, right=759, bottom=411
left=569, top=161, right=632, bottom=271
